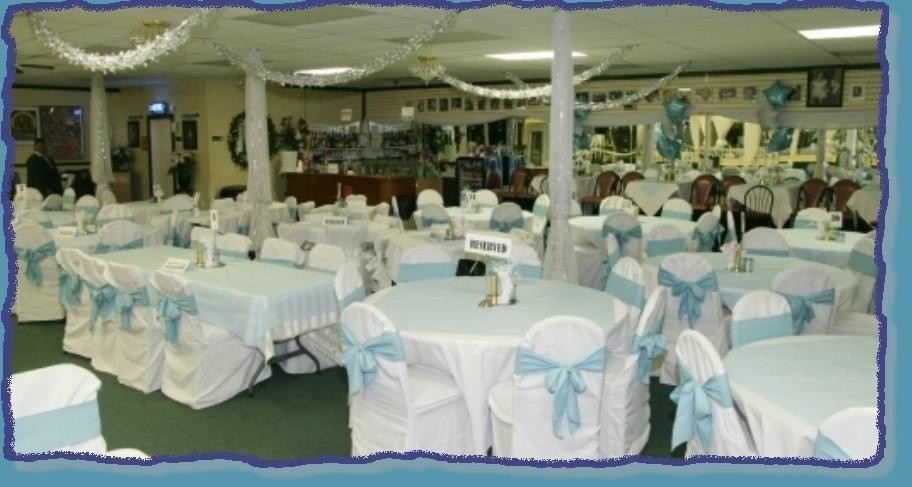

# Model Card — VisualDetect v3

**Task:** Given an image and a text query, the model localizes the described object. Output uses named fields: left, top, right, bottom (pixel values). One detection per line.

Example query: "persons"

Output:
left=809, top=71, right=829, bottom=106
left=25, top=138, right=65, bottom=201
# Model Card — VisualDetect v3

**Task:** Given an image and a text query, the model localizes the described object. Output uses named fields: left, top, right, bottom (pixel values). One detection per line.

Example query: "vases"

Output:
left=179, top=174, right=191, bottom=193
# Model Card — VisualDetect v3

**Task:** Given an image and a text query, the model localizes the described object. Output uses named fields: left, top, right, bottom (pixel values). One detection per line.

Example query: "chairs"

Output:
left=742, top=227, right=789, bottom=257
left=709, top=176, right=747, bottom=214
left=600, top=195, right=627, bottom=214
left=420, top=202, right=449, bottom=226
left=15, top=186, right=43, bottom=203
left=581, top=170, right=620, bottom=212
left=662, top=197, right=693, bottom=223
left=14, top=224, right=68, bottom=323
left=476, top=190, right=498, bottom=206
left=190, top=224, right=213, bottom=250
left=417, top=189, right=443, bottom=207
left=154, top=269, right=271, bottom=410
left=63, top=187, right=75, bottom=211
left=569, top=198, right=582, bottom=218
left=288, top=196, right=298, bottom=216
left=109, top=262, right=166, bottom=395
left=689, top=174, right=717, bottom=215
left=17, top=211, right=50, bottom=227
left=499, top=166, right=531, bottom=198
left=160, top=193, right=193, bottom=214
left=217, top=233, right=252, bottom=259
left=618, top=171, right=644, bottom=195
left=75, top=195, right=101, bottom=228
left=336, top=303, right=473, bottom=456
left=260, top=237, right=304, bottom=268
left=306, top=245, right=349, bottom=274
left=645, top=223, right=687, bottom=254
left=604, top=256, right=647, bottom=355
left=746, top=184, right=774, bottom=234
left=345, top=195, right=368, bottom=207
left=690, top=204, right=722, bottom=252
left=533, top=195, right=552, bottom=234
left=601, top=211, right=642, bottom=267
left=79, top=253, right=121, bottom=376
left=396, top=243, right=458, bottom=282
left=10, top=364, right=146, bottom=460
left=796, top=179, right=831, bottom=214
left=669, top=328, right=752, bottom=458
left=601, top=285, right=670, bottom=459
left=731, top=287, right=795, bottom=347
left=41, top=193, right=63, bottom=211
left=297, top=199, right=316, bottom=221
left=487, top=315, right=608, bottom=458
left=490, top=201, right=523, bottom=234
left=826, top=179, right=861, bottom=226
left=484, top=246, right=546, bottom=278
left=55, top=247, right=92, bottom=359
left=847, top=238, right=879, bottom=316
left=794, top=207, right=831, bottom=230
left=334, top=262, right=365, bottom=313
left=772, top=266, right=843, bottom=336
left=605, top=230, right=620, bottom=266
left=813, top=406, right=878, bottom=459
left=96, top=220, right=144, bottom=251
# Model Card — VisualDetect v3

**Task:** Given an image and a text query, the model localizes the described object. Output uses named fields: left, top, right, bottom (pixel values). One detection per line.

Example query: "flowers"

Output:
left=166, top=151, right=196, bottom=173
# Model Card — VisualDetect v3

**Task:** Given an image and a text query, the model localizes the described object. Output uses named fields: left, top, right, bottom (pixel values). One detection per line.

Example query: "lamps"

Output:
left=408, top=54, right=445, bottom=86
left=130, top=19, right=170, bottom=49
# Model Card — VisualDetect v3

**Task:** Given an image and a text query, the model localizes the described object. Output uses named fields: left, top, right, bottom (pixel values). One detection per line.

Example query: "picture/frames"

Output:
left=11, top=107, right=41, bottom=146
left=806, top=64, right=844, bottom=108
